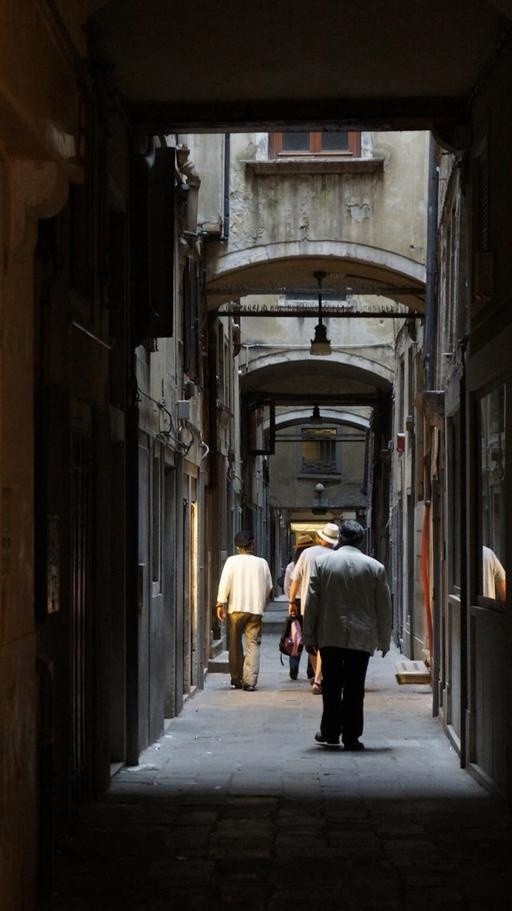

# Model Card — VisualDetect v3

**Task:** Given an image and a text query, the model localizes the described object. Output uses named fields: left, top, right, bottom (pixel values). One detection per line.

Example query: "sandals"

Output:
left=310, top=677, right=322, bottom=694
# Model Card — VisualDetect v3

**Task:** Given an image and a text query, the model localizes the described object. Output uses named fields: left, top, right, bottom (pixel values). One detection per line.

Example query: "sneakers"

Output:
left=243, top=686, right=256, bottom=691
left=231, top=679, right=241, bottom=689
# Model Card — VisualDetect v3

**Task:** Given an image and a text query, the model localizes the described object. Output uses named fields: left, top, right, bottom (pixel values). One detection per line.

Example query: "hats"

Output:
left=317, top=523, right=339, bottom=544
left=293, top=534, right=314, bottom=547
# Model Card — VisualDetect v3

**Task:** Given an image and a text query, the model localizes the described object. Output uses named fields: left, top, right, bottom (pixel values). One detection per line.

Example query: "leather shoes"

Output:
left=316, top=732, right=340, bottom=745
left=345, top=742, right=364, bottom=749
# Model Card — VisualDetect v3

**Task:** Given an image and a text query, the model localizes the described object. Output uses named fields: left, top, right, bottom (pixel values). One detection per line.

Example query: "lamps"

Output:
left=310, top=270, right=333, bottom=356
left=309, top=403, right=323, bottom=423
left=311, top=482, right=329, bottom=515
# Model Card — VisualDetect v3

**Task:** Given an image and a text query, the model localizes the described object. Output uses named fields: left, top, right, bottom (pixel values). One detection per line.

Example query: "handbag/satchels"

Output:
left=278, top=615, right=304, bottom=657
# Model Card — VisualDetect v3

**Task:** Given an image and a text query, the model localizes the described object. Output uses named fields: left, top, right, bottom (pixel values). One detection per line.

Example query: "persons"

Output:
left=479, top=543, right=506, bottom=600
left=288, top=522, right=343, bottom=696
left=300, top=518, right=393, bottom=751
left=213, top=529, right=273, bottom=692
left=283, top=534, right=316, bottom=681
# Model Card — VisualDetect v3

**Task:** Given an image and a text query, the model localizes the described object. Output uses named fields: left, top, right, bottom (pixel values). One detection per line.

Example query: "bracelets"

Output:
left=289, top=601, right=296, bottom=604
left=215, top=604, right=223, bottom=607
